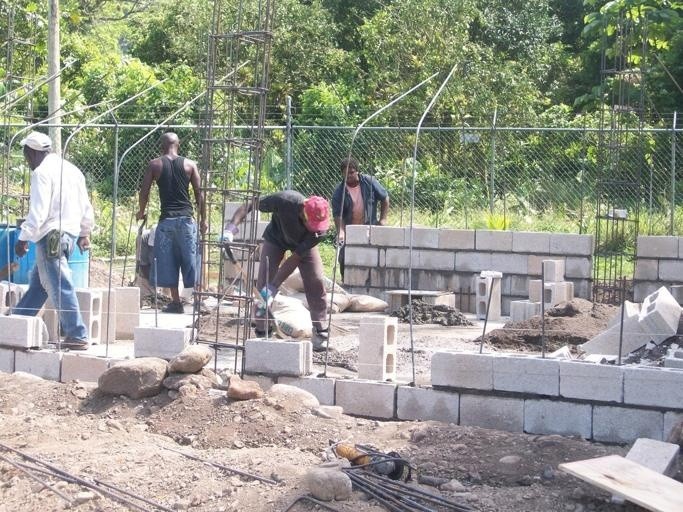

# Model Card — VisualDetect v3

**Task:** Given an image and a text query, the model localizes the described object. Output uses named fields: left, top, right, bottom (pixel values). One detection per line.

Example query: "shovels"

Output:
left=225, top=244, right=291, bottom=340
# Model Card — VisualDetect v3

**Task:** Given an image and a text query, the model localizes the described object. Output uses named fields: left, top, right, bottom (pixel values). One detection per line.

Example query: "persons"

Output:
left=332, top=156, right=390, bottom=280
left=12, top=131, right=94, bottom=351
left=135, top=132, right=207, bottom=316
left=220, top=189, right=331, bottom=340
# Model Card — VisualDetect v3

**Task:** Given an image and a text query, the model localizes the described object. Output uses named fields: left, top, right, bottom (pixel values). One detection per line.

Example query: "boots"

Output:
left=311, top=326, right=334, bottom=352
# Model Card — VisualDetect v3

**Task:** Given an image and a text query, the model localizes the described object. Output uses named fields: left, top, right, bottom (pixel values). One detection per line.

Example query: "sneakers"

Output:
left=194, top=302, right=210, bottom=313
left=57, top=337, right=89, bottom=351
left=162, top=301, right=185, bottom=313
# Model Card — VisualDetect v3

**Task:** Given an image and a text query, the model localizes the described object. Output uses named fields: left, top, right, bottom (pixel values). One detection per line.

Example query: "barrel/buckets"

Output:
left=11, top=218, right=90, bottom=291
left=0, top=224, right=20, bottom=283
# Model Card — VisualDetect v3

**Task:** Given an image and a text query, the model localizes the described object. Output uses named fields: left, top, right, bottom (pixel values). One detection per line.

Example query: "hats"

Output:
left=19, top=131, right=52, bottom=153
left=148, top=223, right=159, bottom=247
left=303, top=195, right=330, bottom=233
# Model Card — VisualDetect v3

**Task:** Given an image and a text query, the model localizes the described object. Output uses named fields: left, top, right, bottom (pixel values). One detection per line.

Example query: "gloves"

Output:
left=220, top=221, right=240, bottom=260
left=254, top=283, right=279, bottom=317
